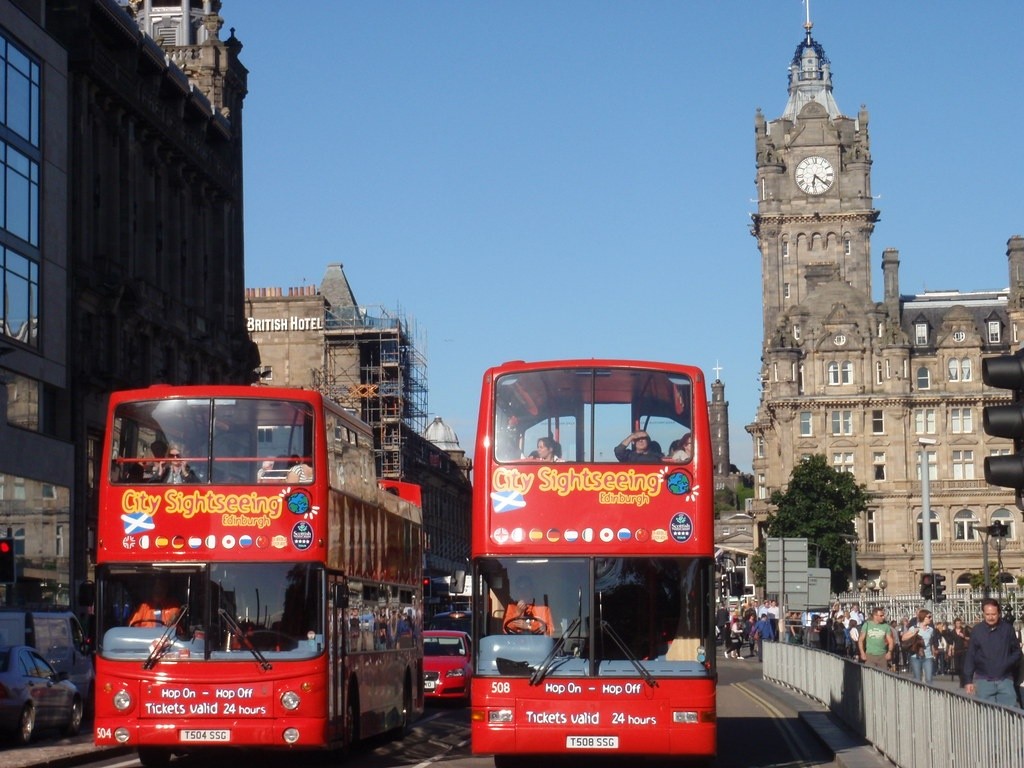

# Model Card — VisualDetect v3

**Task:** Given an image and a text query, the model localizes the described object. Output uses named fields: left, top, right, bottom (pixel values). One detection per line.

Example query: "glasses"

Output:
left=925, top=616, right=932, bottom=620
left=169, top=453, right=182, bottom=459
left=875, top=614, right=884, bottom=617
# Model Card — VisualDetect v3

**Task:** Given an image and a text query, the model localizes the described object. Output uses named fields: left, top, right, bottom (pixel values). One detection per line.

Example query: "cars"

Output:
left=0, top=644, right=83, bottom=747
left=420, top=630, right=472, bottom=697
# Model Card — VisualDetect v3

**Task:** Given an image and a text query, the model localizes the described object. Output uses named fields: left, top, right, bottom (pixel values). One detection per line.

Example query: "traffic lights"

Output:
left=980, top=348, right=1024, bottom=499
left=0, top=536, right=17, bottom=585
left=934, top=574, right=947, bottom=603
left=920, top=573, right=934, bottom=598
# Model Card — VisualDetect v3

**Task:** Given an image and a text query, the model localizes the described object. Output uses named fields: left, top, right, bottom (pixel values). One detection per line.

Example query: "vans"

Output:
left=0, top=611, right=95, bottom=716
left=429, top=611, right=482, bottom=635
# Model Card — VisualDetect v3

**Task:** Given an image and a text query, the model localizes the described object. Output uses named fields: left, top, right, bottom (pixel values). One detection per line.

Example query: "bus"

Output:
left=447, top=360, right=745, bottom=768
left=77, top=386, right=425, bottom=768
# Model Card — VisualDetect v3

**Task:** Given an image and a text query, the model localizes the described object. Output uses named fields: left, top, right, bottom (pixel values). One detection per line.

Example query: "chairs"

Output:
left=270, top=455, right=289, bottom=477
left=667, top=441, right=680, bottom=453
left=126, top=464, right=144, bottom=482
left=554, top=442, right=561, bottom=456
left=633, top=440, right=658, bottom=457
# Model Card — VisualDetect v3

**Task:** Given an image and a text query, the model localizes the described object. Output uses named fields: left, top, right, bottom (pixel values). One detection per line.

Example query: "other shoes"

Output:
left=724, top=650, right=745, bottom=659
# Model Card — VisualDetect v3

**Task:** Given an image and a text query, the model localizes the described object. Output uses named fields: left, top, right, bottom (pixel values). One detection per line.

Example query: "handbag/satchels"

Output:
left=902, top=626, right=924, bottom=654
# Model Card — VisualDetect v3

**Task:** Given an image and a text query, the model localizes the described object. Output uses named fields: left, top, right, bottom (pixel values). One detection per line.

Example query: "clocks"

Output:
left=794, top=156, right=835, bottom=195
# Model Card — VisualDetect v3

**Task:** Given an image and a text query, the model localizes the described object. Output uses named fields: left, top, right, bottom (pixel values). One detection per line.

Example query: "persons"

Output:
left=714, top=597, right=1024, bottom=759
left=148, top=442, right=313, bottom=484
left=502, top=575, right=553, bottom=636
left=524, top=430, right=692, bottom=462
left=128, top=577, right=184, bottom=641
